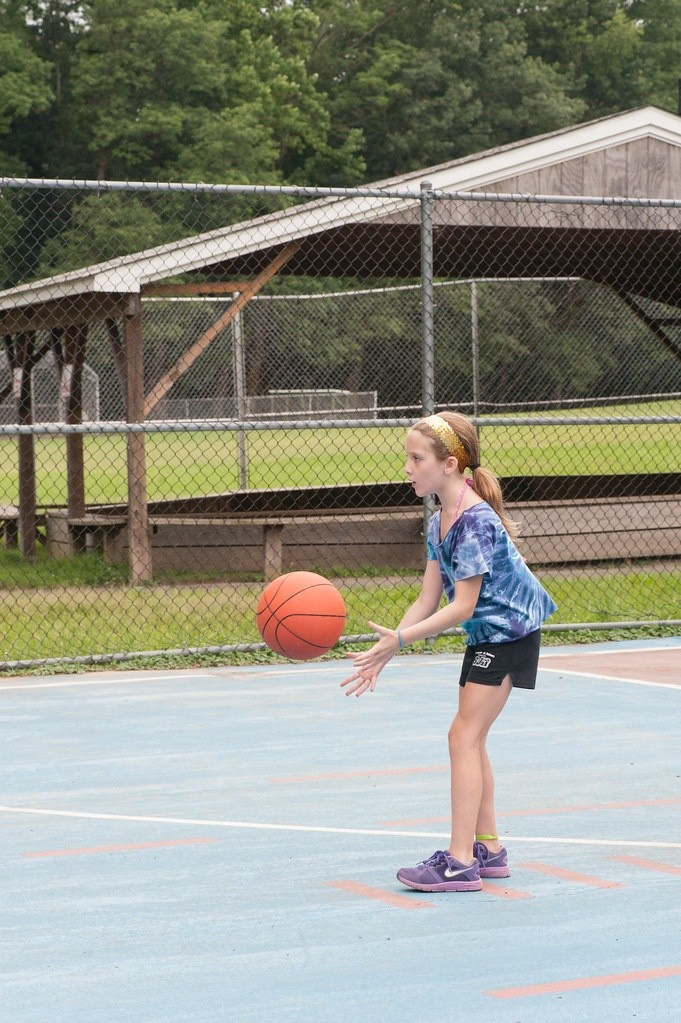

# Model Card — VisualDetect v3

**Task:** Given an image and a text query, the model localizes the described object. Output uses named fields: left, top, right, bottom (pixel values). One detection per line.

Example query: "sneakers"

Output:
left=396, top=849, right=483, bottom=893
left=473, top=842, right=510, bottom=878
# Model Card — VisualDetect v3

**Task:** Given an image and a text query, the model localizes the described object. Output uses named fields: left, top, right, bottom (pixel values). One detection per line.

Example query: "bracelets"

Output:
left=398, top=630, right=403, bottom=648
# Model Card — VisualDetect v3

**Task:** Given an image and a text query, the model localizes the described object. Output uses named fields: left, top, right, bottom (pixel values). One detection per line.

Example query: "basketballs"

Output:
left=258, top=570, right=345, bottom=659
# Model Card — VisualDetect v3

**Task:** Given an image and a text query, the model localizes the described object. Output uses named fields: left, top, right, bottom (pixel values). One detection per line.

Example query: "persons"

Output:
left=339, top=412, right=558, bottom=891
